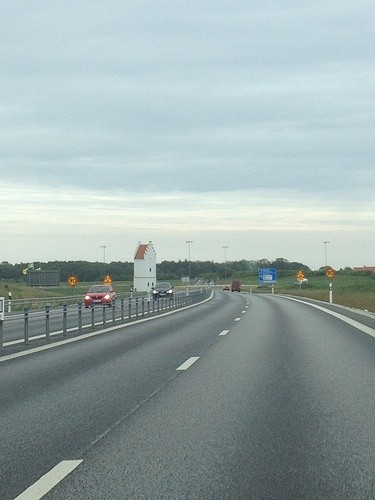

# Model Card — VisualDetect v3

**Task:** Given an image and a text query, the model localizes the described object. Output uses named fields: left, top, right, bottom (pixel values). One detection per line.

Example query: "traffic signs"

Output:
left=259, top=267, right=277, bottom=283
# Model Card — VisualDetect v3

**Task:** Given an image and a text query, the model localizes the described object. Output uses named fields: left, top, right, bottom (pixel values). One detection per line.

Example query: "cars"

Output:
left=84, top=284, right=116, bottom=308
left=223, top=286, right=230, bottom=291
left=152, top=283, right=175, bottom=300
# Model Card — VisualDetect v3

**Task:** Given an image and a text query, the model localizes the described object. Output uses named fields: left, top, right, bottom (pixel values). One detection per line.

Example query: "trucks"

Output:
left=231, top=281, right=241, bottom=292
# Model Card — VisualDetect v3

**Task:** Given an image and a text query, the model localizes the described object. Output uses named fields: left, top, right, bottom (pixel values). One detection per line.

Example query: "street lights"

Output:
left=222, top=246, right=229, bottom=284
left=186, top=241, right=193, bottom=275
left=100, top=246, right=106, bottom=264
left=325, top=241, right=330, bottom=265
